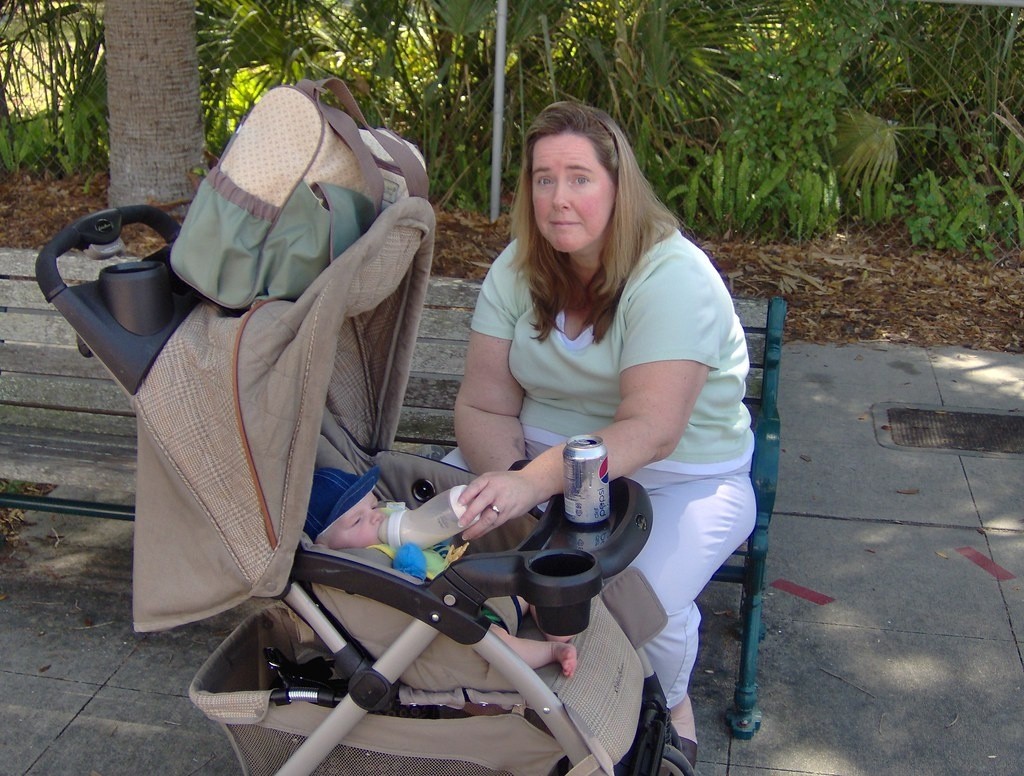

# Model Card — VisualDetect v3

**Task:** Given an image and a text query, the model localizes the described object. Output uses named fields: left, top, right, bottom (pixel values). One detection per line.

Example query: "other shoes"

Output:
left=670, top=735, right=698, bottom=776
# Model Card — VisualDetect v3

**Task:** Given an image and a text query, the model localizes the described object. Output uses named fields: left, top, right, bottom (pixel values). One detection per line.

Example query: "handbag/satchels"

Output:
left=171, top=78, right=429, bottom=313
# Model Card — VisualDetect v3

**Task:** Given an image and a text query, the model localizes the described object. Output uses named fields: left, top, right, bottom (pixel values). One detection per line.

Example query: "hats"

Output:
left=303, top=465, right=381, bottom=543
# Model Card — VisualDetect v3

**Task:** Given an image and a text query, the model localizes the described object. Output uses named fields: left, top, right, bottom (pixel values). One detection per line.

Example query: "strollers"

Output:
left=36, top=202, right=694, bottom=776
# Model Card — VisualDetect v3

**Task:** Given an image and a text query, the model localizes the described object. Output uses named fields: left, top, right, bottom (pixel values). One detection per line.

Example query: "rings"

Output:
left=489, top=504, right=500, bottom=516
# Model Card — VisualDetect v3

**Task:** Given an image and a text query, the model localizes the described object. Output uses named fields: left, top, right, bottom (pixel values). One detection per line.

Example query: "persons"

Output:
left=436, top=101, right=757, bottom=776
left=301, top=465, right=577, bottom=692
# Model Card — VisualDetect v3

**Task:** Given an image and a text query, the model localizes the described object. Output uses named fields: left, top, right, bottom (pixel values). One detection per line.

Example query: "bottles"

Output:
left=378, top=485, right=482, bottom=551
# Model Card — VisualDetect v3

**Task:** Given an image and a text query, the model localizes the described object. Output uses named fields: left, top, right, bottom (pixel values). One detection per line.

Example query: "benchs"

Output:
left=0, top=248, right=784, bottom=738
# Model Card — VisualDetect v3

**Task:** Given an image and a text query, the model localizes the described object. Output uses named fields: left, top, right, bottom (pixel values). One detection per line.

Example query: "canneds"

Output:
left=562, top=435, right=609, bottom=524
